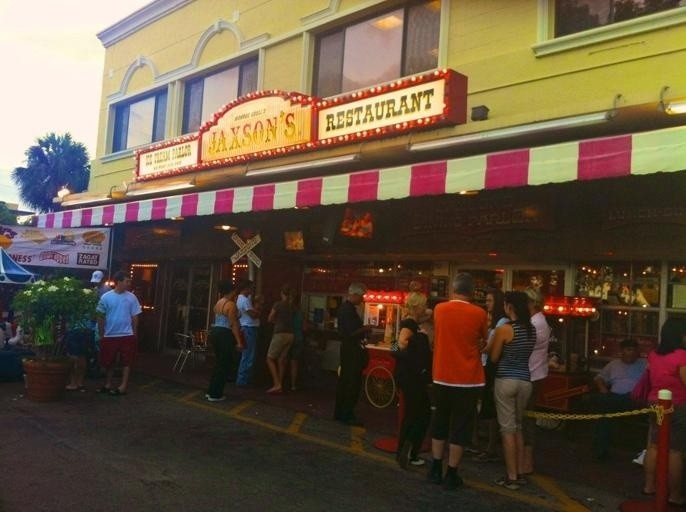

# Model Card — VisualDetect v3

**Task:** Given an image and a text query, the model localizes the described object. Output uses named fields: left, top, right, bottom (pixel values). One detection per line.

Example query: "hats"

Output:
left=525, top=287, right=543, bottom=306
left=90, top=270, right=105, bottom=284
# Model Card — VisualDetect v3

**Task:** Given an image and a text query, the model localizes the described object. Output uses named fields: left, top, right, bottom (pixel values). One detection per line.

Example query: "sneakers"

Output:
left=408, top=456, right=424, bottom=466
left=494, top=472, right=520, bottom=490
left=204, top=393, right=227, bottom=402
left=398, top=440, right=412, bottom=469
left=516, top=474, right=528, bottom=485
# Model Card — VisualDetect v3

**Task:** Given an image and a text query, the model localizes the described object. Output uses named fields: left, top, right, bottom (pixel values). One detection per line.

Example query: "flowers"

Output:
left=9, top=276, right=99, bottom=360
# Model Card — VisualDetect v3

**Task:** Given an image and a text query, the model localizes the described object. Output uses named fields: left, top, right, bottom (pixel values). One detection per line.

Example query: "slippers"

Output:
left=107, top=388, right=126, bottom=395
left=94, top=386, right=113, bottom=393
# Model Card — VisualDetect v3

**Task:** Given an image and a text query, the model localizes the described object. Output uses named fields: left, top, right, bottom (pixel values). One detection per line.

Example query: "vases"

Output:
left=22, top=357, right=65, bottom=401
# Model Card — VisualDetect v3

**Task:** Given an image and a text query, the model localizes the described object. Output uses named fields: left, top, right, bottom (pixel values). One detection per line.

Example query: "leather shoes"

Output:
left=335, top=415, right=363, bottom=426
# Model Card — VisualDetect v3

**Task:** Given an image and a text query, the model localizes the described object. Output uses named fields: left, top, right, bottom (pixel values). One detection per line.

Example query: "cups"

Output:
left=55, top=232, right=61, bottom=241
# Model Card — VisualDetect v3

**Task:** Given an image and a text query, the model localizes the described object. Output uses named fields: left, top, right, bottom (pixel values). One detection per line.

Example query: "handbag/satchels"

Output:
left=351, top=336, right=368, bottom=368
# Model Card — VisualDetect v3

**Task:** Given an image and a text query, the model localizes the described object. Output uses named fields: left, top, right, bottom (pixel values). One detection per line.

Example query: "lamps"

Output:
left=244, top=142, right=367, bottom=177
left=125, top=174, right=196, bottom=197
left=406, top=95, right=619, bottom=149
left=60, top=186, right=115, bottom=206
left=657, top=87, right=685, bottom=115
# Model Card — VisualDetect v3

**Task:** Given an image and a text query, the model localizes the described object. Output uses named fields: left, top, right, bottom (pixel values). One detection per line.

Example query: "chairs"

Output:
left=192, top=332, right=209, bottom=366
left=173, top=332, right=200, bottom=372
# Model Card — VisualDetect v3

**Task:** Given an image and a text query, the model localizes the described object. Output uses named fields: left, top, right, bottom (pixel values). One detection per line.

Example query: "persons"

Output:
left=342, top=206, right=373, bottom=239
left=333, top=281, right=371, bottom=426
left=0, top=269, right=144, bottom=397
left=582, top=337, right=648, bottom=473
left=293, top=232, right=304, bottom=248
left=640, top=316, right=686, bottom=509
left=204, top=274, right=324, bottom=403
left=394, top=271, right=552, bottom=491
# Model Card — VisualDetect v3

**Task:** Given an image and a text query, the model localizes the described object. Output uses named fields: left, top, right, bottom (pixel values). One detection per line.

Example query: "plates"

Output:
left=80, top=229, right=107, bottom=245
